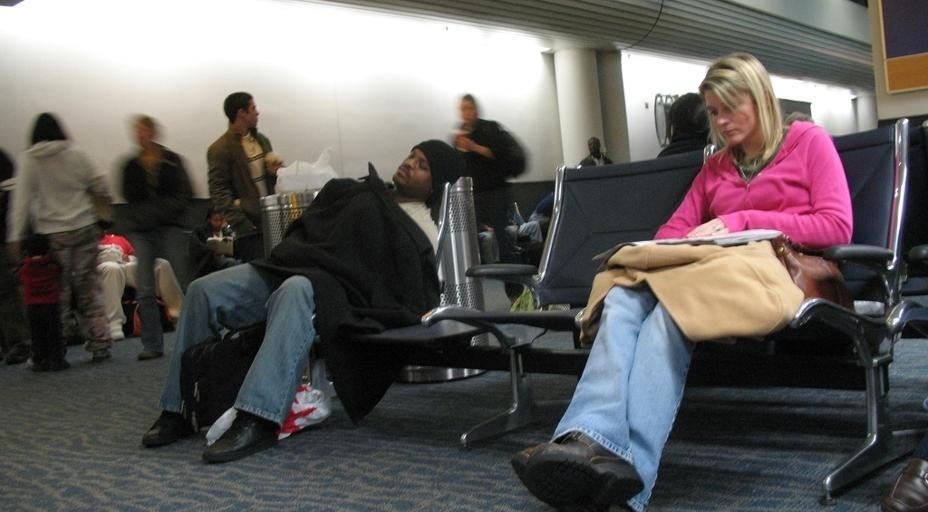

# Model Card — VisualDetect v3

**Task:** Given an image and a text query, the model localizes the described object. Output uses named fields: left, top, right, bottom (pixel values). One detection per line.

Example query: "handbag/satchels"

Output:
left=773, top=235, right=857, bottom=311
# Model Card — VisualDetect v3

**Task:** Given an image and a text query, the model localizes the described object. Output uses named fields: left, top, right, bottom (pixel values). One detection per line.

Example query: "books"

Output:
left=592, top=227, right=783, bottom=268
left=203, top=237, right=235, bottom=256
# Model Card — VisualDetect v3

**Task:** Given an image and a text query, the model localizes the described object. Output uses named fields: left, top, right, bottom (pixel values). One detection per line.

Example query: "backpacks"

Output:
left=484, top=125, right=527, bottom=180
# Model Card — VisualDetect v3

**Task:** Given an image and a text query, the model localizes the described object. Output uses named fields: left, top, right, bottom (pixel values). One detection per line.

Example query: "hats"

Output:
left=411, top=140, right=457, bottom=192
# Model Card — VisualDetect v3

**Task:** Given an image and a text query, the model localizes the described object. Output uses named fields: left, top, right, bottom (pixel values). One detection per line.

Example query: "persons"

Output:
left=579, top=136, right=613, bottom=165
left=121, top=113, right=194, bottom=361
left=142, top=140, right=457, bottom=464
left=0, top=149, right=32, bottom=365
left=16, top=235, right=59, bottom=367
left=454, top=95, right=518, bottom=296
left=206, top=92, right=287, bottom=260
left=657, top=93, right=709, bottom=158
left=7, top=114, right=115, bottom=369
left=191, top=208, right=242, bottom=276
left=96, top=231, right=185, bottom=341
left=510, top=52, right=852, bottom=512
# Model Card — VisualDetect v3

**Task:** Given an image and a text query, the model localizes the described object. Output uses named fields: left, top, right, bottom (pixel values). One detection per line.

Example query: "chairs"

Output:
left=422, top=116, right=922, bottom=507
left=900, top=127, right=927, bottom=296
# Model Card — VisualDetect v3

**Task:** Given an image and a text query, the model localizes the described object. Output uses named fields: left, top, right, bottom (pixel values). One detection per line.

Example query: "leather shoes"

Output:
left=203, top=414, right=277, bottom=462
left=93, top=352, right=111, bottom=362
left=512, top=448, right=632, bottom=512
left=531, top=434, right=643, bottom=503
left=138, top=350, right=163, bottom=361
left=143, top=412, right=191, bottom=447
